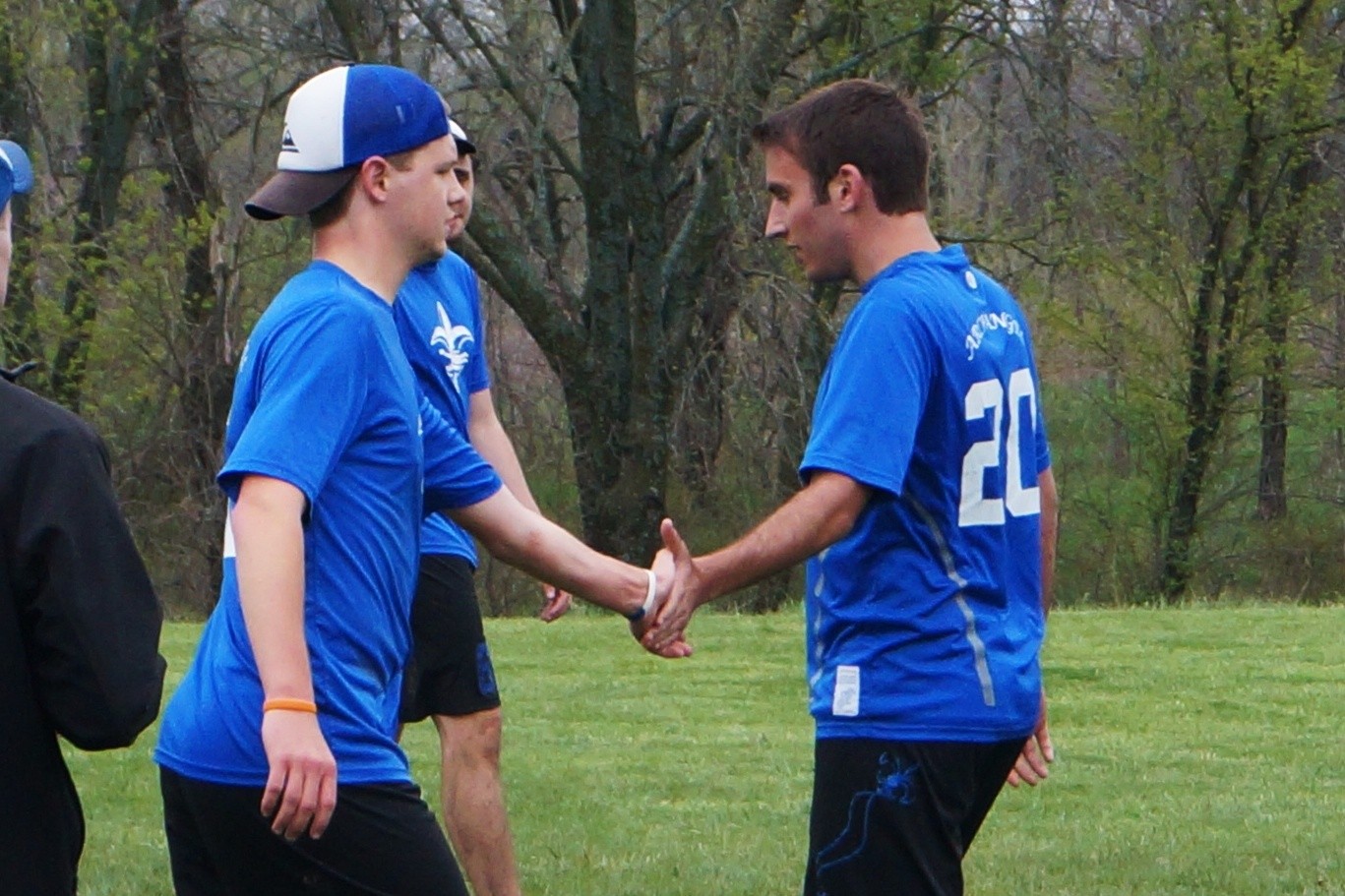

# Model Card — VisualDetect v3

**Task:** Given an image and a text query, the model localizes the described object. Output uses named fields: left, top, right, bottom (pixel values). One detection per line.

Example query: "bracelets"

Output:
left=624, top=569, right=656, bottom=621
left=264, top=700, right=317, bottom=714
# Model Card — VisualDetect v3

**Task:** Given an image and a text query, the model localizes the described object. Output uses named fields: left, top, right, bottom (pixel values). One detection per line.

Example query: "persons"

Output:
left=646, top=80, right=1054, bottom=895
left=0, top=139, right=167, bottom=896
left=393, top=119, right=572, bottom=895
left=150, top=64, right=692, bottom=896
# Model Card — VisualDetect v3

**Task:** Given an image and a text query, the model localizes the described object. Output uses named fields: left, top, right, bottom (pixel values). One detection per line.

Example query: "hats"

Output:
left=243, top=66, right=447, bottom=222
left=444, top=114, right=477, bottom=157
left=0, top=139, right=33, bottom=214
